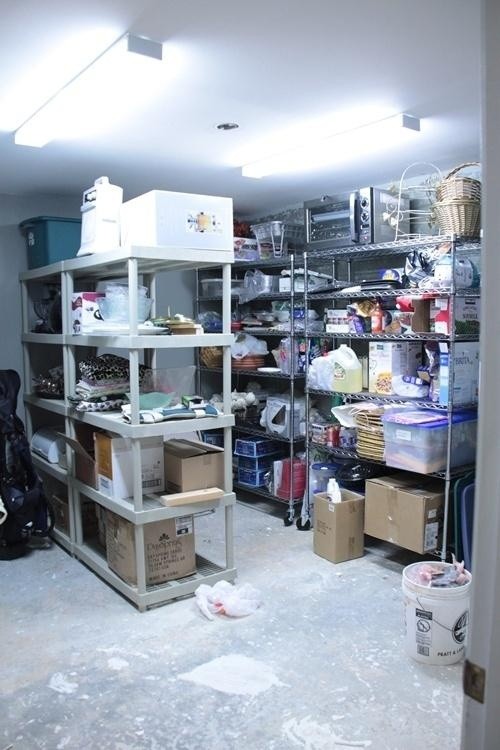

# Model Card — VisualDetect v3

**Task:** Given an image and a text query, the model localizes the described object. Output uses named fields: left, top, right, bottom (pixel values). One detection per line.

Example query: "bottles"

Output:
left=311, top=423, right=356, bottom=449
left=371, top=297, right=386, bottom=334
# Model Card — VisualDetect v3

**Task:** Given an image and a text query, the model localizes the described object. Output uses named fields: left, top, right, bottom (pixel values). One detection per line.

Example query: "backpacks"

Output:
left=0, top=368, right=56, bottom=561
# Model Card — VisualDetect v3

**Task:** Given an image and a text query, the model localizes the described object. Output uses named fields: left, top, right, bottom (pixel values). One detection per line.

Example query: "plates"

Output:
left=257, top=367, right=281, bottom=372
left=93, top=327, right=168, bottom=335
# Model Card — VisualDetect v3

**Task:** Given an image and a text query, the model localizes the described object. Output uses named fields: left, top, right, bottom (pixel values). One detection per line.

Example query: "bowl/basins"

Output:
left=96, top=281, right=155, bottom=324
left=336, top=460, right=385, bottom=491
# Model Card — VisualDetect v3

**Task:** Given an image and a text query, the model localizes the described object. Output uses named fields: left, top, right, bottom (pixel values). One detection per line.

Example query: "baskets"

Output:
left=432, top=162, right=481, bottom=235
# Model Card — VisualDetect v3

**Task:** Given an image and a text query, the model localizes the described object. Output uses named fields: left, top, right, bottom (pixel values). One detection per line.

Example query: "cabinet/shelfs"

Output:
left=64, top=249, right=237, bottom=612
left=18, top=260, right=74, bottom=554
left=303, top=235, right=480, bottom=562
left=192, top=255, right=338, bottom=525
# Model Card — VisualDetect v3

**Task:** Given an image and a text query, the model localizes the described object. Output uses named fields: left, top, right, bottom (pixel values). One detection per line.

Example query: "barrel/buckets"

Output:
left=332, top=343, right=364, bottom=393
left=401, top=560, right=472, bottom=667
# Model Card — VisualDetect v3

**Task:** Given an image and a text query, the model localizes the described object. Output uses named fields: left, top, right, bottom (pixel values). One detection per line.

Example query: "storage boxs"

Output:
left=381, top=411, right=475, bottom=475
left=396, top=296, right=480, bottom=336
left=17, top=216, right=82, bottom=270
left=161, top=437, right=225, bottom=491
left=70, top=291, right=105, bottom=330
left=54, top=494, right=100, bottom=541
left=199, top=279, right=243, bottom=299
left=440, top=344, right=479, bottom=405
left=327, top=309, right=371, bottom=333
left=106, top=510, right=198, bottom=587
left=265, top=398, right=315, bottom=439
left=313, top=487, right=366, bottom=565
left=58, top=431, right=94, bottom=488
left=370, top=341, right=422, bottom=399
left=234, top=437, right=288, bottom=488
left=361, top=479, right=454, bottom=554
left=119, top=187, right=234, bottom=250
left=94, top=434, right=167, bottom=500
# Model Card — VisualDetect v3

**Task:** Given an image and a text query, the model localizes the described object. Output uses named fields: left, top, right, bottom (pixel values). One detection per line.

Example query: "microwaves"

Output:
left=303, top=184, right=410, bottom=253
